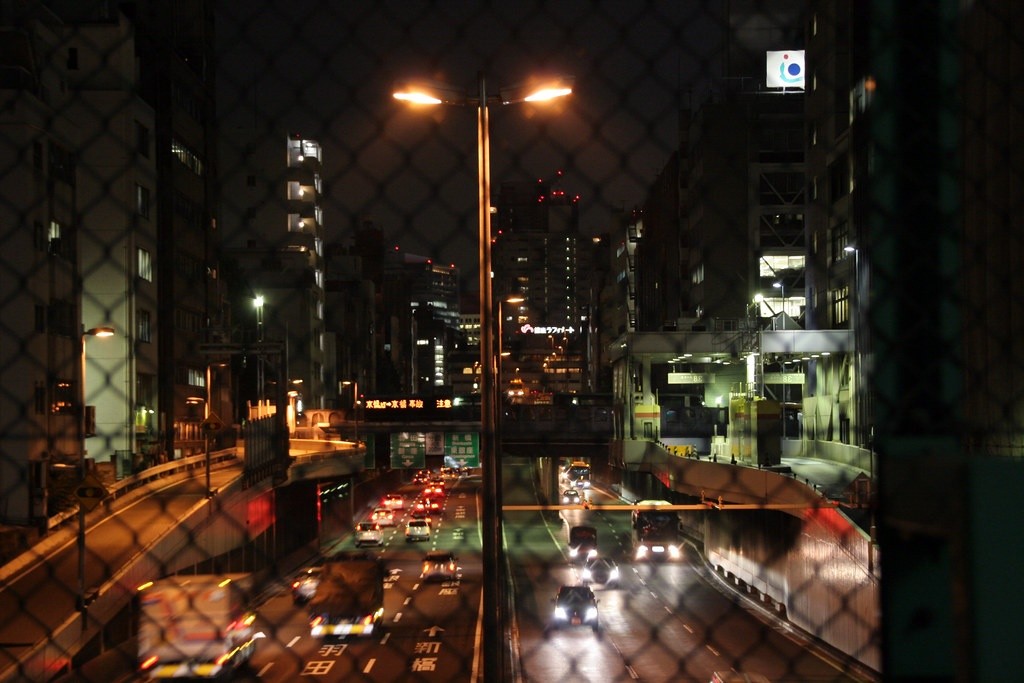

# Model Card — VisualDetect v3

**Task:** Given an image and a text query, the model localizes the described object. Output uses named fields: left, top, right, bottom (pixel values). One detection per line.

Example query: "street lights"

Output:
left=496, top=294, right=524, bottom=520
left=73, top=322, right=358, bottom=588
left=772, top=282, right=786, bottom=438
left=393, top=75, right=572, bottom=683
left=844, top=247, right=859, bottom=293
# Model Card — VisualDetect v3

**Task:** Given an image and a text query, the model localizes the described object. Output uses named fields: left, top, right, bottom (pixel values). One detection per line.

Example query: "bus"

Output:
left=569, top=461, right=592, bottom=489
left=631, top=500, right=681, bottom=562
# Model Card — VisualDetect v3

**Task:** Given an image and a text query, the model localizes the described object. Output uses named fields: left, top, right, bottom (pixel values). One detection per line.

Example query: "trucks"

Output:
left=137, top=560, right=383, bottom=683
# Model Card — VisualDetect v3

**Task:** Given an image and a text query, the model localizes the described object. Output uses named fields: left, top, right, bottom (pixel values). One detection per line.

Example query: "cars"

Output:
left=291, top=568, right=320, bottom=601
left=567, top=524, right=619, bottom=589
left=354, top=522, right=384, bottom=547
left=559, top=459, right=567, bottom=472
left=385, top=494, right=405, bottom=510
left=551, top=585, right=600, bottom=632
left=405, top=519, right=430, bottom=541
left=562, top=490, right=580, bottom=504
left=508, top=383, right=525, bottom=397
left=422, top=550, right=458, bottom=581
left=411, top=465, right=459, bottom=517
left=372, top=508, right=396, bottom=526
